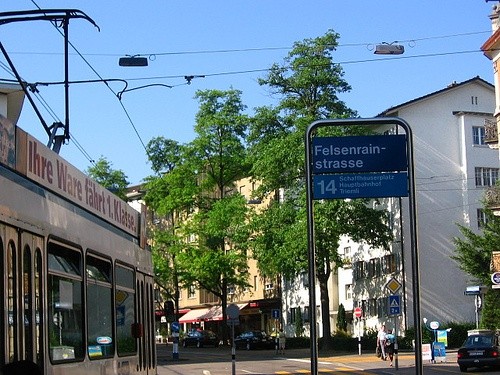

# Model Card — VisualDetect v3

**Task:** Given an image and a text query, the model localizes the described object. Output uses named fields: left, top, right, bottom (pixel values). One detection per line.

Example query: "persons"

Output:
left=279, top=329, right=285, bottom=355
left=384, top=330, right=395, bottom=366
left=377, top=325, right=387, bottom=360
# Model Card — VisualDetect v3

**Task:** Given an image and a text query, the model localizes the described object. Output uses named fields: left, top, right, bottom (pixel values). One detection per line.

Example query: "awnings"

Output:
left=178, top=303, right=249, bottom=323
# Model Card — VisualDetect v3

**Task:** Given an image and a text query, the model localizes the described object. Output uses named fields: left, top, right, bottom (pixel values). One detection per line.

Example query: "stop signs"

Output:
left=354, top=307, right=362, bottom=317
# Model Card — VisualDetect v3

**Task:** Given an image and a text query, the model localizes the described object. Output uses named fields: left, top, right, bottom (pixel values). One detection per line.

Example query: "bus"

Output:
left=0, top=8, right=176, bottom=375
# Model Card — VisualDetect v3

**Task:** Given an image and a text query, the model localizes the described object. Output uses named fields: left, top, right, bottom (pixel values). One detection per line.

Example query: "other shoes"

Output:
left=390, top=363, right=393, bottom=366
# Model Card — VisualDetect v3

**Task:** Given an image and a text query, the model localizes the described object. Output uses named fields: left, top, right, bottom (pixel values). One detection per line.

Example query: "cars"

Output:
left=182, top=327, right=224, bottom=349
left=230, top=329, right=289, bottom=351
left=457, top=333, right=500, bottom=372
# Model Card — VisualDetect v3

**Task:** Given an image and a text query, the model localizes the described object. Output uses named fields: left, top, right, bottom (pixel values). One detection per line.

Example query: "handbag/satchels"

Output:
left=376, top=347, right=381, bottom=358
